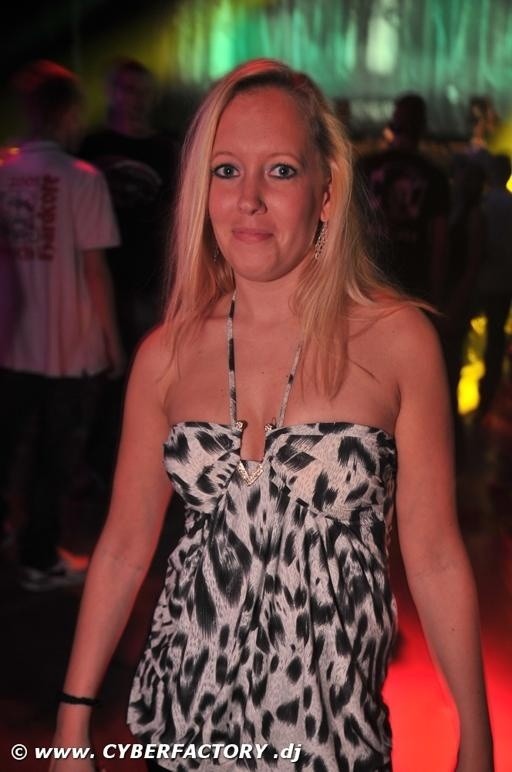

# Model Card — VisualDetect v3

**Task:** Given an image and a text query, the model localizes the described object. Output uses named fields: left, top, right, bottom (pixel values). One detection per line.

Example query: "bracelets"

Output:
left=58, top=692, right=96, bottom=708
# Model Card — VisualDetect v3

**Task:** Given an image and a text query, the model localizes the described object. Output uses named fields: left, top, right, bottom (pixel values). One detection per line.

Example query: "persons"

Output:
left=1, top=61, right=510, bottom=589
left=48, top=59, right=494, bottom=772
